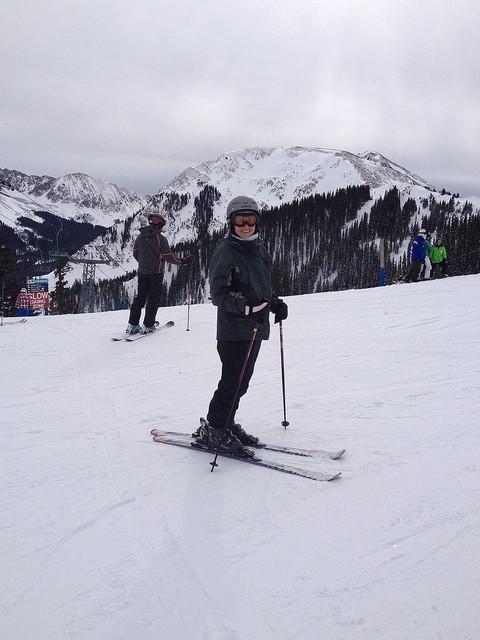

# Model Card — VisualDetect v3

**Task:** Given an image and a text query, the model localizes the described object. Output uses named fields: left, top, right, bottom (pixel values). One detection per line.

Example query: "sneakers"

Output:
left=144, top=326, right=156, bottom=333
left=232, top=424, right=259, bottom=444
left=208, top=429, right=243, bottom=449
left=130, top=325, right=142, bottom=334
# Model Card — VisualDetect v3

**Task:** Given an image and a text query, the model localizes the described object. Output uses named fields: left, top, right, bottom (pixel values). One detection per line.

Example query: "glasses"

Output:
left=230, top=211, right=261, bottom=227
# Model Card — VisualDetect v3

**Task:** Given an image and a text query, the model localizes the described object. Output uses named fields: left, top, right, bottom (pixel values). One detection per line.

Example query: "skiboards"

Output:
left=111, top=320, right=175, bottom=341
left=1, top=318, right=27, bottom=327
left=149, top=427, right=346, bottom=483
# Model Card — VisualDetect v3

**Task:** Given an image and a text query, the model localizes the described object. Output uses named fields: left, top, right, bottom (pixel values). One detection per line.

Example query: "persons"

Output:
left=188, top=193, right=289, bottom=454
left=428, top=236, right=449, bottom=280
left=125, top=213, right=195, bottom=336
left=416, top=234, right=435, bottom=282
left=402, top=229, right=427, bottom=284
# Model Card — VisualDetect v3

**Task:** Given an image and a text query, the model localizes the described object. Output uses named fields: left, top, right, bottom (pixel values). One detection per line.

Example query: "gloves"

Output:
left=271, top=295, right=288, bottom=324
left=244, top=298, right=269, bottom=316
left=181, top=254, right=195, bottom=265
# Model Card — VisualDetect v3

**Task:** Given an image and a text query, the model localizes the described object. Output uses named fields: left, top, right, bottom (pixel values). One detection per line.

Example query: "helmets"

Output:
left=227, top=196, right=258, bottom=218
left=148, top=214, right=166, bottom=225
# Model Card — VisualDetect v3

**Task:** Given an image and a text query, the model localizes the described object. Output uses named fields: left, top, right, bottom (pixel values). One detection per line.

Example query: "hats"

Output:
left=419, top=229, right=426, bottom=232
